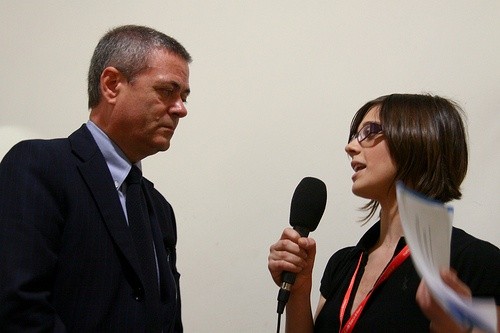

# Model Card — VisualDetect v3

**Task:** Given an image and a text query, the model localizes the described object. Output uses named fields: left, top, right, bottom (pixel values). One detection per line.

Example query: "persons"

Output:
left=268, top=92, right=500, bottom=332
left=0, top=24, right=194, bottom=332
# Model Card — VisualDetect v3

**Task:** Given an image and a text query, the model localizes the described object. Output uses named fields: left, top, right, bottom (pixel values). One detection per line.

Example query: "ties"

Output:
left=122, top=165, right=159, bottom=306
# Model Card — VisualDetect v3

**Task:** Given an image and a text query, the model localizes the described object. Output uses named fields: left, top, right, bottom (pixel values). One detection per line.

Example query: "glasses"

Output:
left=349, top=123, right=382, bottom=143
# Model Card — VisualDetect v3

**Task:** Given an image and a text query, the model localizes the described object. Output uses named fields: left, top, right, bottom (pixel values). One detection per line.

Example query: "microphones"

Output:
left=277, top=177, right=327, bottom=313
